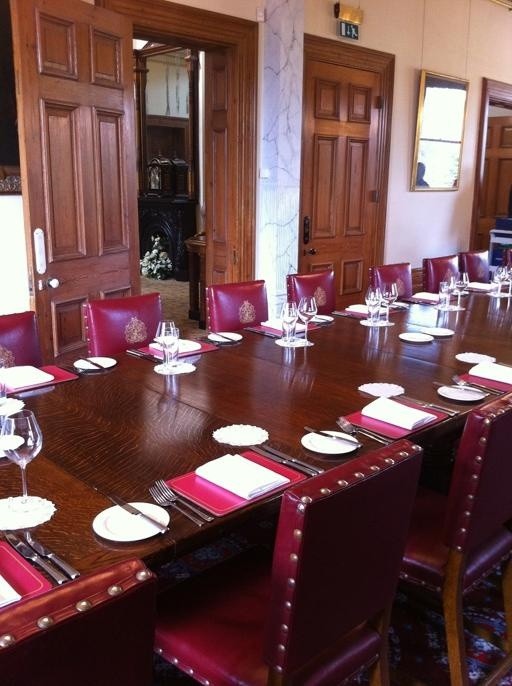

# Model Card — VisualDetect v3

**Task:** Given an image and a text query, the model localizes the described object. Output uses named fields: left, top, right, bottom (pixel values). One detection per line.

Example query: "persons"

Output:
left=414, top=161, right=429, bottom=189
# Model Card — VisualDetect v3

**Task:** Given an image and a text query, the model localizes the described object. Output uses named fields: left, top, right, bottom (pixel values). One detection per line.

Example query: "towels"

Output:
left=194, top=452, right=292, bottom=501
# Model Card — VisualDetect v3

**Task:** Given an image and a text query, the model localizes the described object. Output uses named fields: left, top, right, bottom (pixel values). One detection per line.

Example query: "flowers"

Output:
left=137, top=232, right=174, bottom=282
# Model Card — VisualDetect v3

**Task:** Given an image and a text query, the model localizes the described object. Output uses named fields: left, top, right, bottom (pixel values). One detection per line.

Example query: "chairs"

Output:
left=0, top=250, right=511, bottom=686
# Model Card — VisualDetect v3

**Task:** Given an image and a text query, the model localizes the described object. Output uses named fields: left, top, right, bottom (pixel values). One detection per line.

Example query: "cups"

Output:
left=284, top=309, right=297, bottom=343
left=0, top=359, right=7, bottom=406
left=438, top=281, right=449, bottom=311
left=496, top=265, right=509, bottom=281
left=367, top=298, right=380, bottom=323
left=164, top=327, right=180, bottom=368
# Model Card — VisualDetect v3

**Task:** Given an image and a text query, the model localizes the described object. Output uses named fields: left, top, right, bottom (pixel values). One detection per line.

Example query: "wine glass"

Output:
left=280, top=301, right=298, bottom=347
left=380, top=282, right=398, bottom=326
left=493, top=270, right=503, bottom=297
left=158, top=320, right=176, bottom=372
left=453, top=272, right=469, bottom=311
left=364, top=287, right=381, bottom=326
left=444, top=275, right=456, bottom=310
left=2, top=409, right=44, bottom=516
left=298, top=297, right=318, bottom=347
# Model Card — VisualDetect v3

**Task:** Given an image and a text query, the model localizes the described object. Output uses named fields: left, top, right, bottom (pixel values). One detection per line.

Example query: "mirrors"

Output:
left=409, top=67, right=474, bottom=193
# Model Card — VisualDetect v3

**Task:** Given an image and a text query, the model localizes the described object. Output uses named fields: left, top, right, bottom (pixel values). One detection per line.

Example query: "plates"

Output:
left=93, top=503, right=172, bottom=545
left=261, top=320, right=306, bottom=334
left=301, top=431, right=359, bottom=455
left=380, top=301, right=410, bottom=309
left=73, top=358, right=117, bottom=371
left=411, top=292, right=440, bottom=302
left=207, top=331, right=243, bottom=344
left=436, top=385, right=487, bottom=402
left=308, top=315, right=335, bottom=323
left=345, top=303, right=388, bottom=316
left=421, top=327, right=455, bottom=337
left=398, top=332, right=434, bottom=344
left=357, top=382, right=407, bottom=397
left=455, top=351, right=497, bottom=364
left=467, top=281, right=493, bottom=291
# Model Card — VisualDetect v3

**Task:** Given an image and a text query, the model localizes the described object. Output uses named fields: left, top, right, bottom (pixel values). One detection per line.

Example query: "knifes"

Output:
left=126, top=348, right=162, bottom=364
left=412, top=399, right=460, bottom=413
left=107, top=493, right=170, bottom=534
left=263, top=443, right=326, bottom=475
left=250, top=445, right=316, bottom=479
left=303, top=426, right=363, bottom=447
left=25, top=531, right=81, bottom=583
left=7, top=531, right=67, bottom=587
left=433, top=382, right=490, bottom=396
left=207, top=329, right=240, bottom=344
left=243, top=327, right=276, bottom=338
left=77, top=354, right=107, bottom=371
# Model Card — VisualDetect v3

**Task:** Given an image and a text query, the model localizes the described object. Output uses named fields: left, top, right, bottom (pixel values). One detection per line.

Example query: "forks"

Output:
left=336, top=421, right=388, bottom=445
left=156, top=479, right=213, bottom=523
left=339, top=416, right=394, bottom=443
left=451, top=375, right=505, bottom=396
left=149, top=487, right=205, bottom=529
